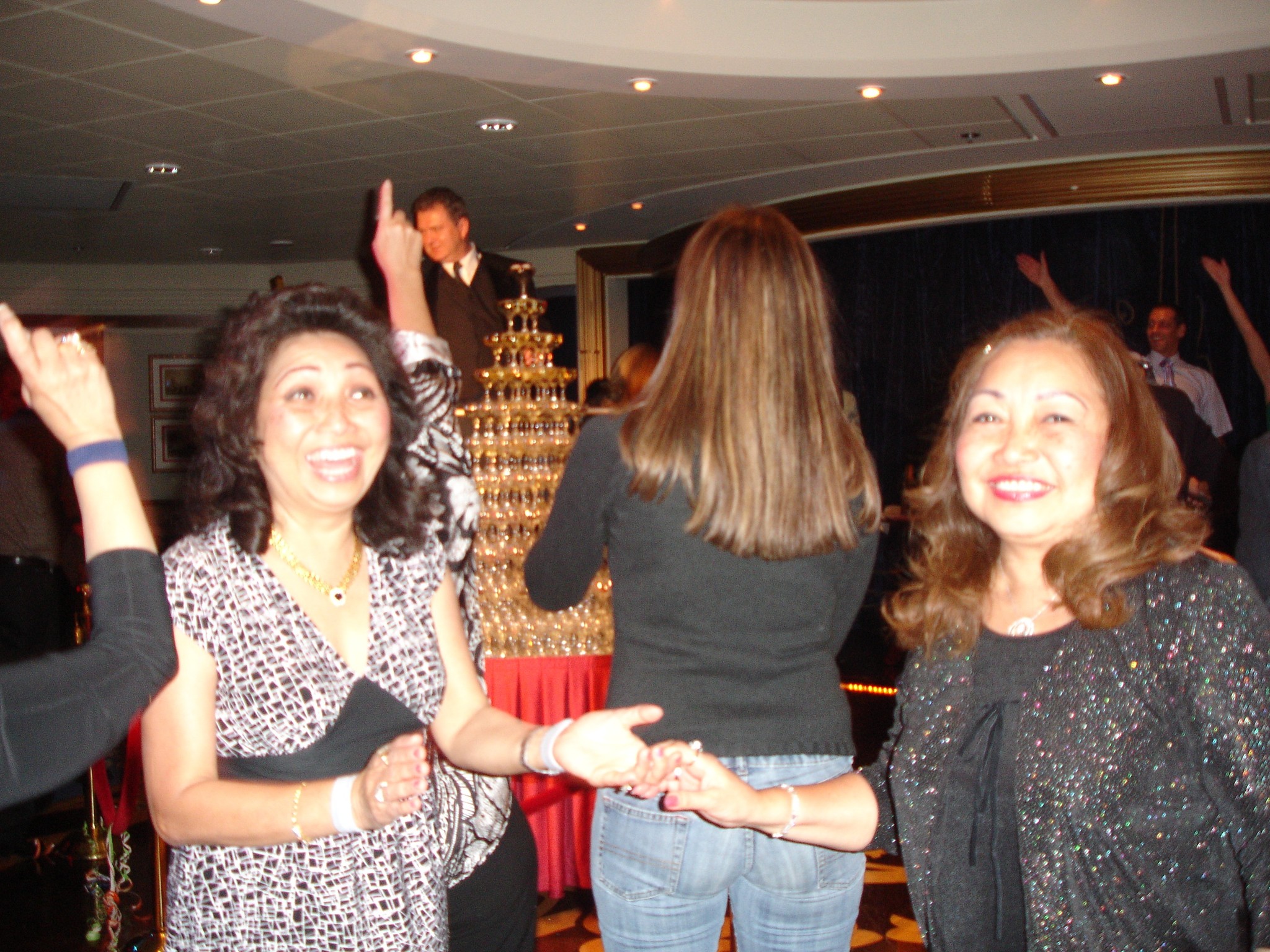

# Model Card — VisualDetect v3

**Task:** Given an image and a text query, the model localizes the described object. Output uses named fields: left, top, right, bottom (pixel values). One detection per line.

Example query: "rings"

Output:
left=380, top=754, right=392, bottom=767
left=618, top=784, right=633, bottom=794
left=689, top=739, right=702, bottom=754
left=375, top=782, right=388, bottom=803
left=57, top=332, right=85, bottom=356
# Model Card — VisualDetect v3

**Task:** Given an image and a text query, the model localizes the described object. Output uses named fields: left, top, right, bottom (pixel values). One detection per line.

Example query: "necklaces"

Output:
left=987, top=580, right=1065, bottom=636
left=268, top=525, right=363, bottom=609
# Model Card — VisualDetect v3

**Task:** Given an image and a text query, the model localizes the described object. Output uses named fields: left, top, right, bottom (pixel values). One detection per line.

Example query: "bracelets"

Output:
left=291, top=781, right=312, bottom=845
left=331, top=776, right=364, bottom=833
left=521, top=725, right=554, bottom=775
left=65, top=441, right=129, bottom=473
left=767, top=783, right=800, bottom=838
left=542, top=719, right=576, bottom=775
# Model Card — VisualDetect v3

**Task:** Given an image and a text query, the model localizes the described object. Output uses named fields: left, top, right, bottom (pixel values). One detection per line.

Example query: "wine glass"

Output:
left=455, top=262, right=613, bottom=657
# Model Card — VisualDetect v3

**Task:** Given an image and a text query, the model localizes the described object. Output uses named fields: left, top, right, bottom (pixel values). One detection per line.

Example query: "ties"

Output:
left=452, top=261, right=468, bottom=288
left=1162, top=359, right=1174, bottom=387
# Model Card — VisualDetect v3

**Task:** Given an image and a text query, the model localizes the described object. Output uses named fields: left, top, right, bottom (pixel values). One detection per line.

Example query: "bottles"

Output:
left=902, top=462, right=916, bottom=511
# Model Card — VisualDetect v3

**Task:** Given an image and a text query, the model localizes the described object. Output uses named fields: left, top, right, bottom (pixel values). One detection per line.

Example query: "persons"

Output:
left=0, top=175, right=1270, bottom=952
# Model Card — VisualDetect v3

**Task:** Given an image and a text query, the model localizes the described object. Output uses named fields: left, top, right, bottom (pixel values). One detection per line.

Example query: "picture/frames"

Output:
left=148, top=352, right=209, bottom=412
left=151, top=416, right=202, bottom=474
